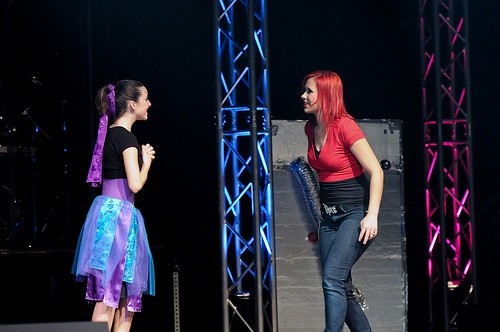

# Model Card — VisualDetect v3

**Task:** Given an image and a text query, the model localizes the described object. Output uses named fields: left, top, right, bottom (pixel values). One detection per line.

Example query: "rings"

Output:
left=370, top=232, right=375, bottom=235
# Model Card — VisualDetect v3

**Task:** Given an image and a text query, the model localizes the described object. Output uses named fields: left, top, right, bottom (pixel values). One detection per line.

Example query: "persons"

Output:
left=300, top=71, right=386, bottom=332
left=72, top=80, right=155, bottom=332
